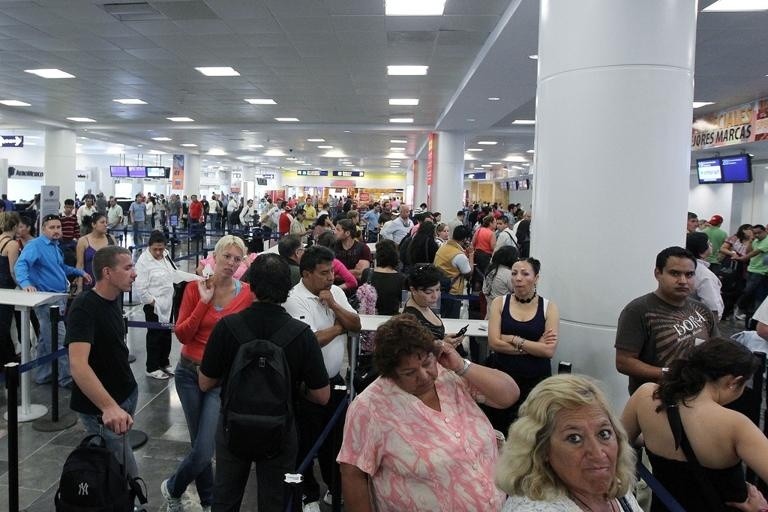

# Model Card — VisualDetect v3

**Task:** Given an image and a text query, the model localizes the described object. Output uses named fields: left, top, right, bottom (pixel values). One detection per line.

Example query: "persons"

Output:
left=1, top=193, right=93, bottom=388
left=64, top=188, right=559, bottom=512
left=615, top=212, right=768, bottom=512
left=493, top=372, right=643, bottom=512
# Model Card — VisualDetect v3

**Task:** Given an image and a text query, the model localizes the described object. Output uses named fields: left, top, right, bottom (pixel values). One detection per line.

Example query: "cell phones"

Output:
left=453, top=324, right=470, bottom=339
left=464, top=239, right=471, bottom=247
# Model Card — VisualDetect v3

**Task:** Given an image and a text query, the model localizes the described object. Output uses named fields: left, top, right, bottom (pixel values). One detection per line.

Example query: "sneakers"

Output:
left=145, top=365, right=176, bottom=380
left=302, top=488, right=344, bottom=512
left=160, top=479, right=183, bottom=512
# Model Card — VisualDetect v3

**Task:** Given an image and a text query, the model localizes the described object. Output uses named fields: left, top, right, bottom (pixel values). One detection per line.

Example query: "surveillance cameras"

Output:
left=289, top=149, right=293, bottom=152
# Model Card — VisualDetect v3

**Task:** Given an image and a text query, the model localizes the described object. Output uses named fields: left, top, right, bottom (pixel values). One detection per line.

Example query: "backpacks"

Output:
left=54, top=434, right=149, bottom=511
left=222, top=313, right=309, bottom=462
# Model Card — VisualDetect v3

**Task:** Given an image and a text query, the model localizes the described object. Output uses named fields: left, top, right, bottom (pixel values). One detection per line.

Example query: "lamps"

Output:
left=261, top=143, right=287, bottom=156
left=463, top=153, right=478, bottom=160
left=205, top=148, right=227, bottom=156
left=501, top=152, right=529, bottom=162
left=320, top=149, right=350, bottom=158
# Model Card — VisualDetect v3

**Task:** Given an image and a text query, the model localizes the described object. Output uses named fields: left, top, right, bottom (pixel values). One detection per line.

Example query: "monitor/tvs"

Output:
left=110, top=166, right=128, bottom=177
left=508, top=181, right=518, bottom=191
left=519, top=179, right=529, bottom=190
left=697, top=157, right=723, bottom=184
left=721, top=154, right=752, bottom=183
left=500, top=182, right=508, bottom=191
left=164, top=167, right=170, bottom=178
left=128, top=166, right=146, bottom=177
left=257, top=177, right=267, bottom=185
left=146, top=166, right=166, bottom=177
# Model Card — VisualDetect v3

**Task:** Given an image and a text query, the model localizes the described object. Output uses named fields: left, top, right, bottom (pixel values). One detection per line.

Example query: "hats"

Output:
left=708, top=215, right=723, bottom=226
